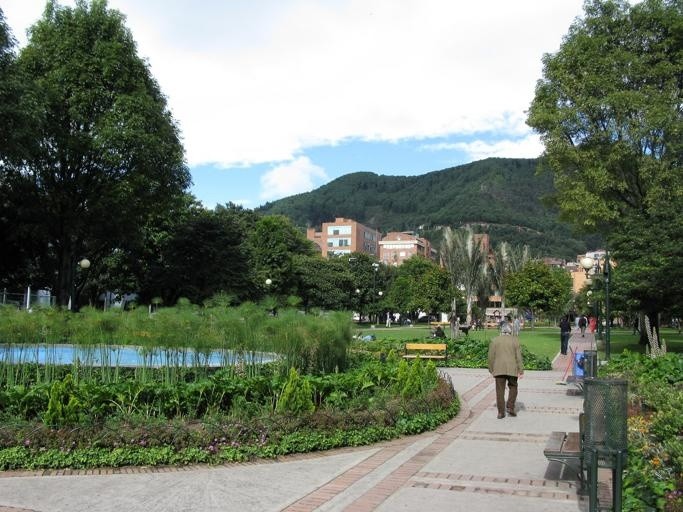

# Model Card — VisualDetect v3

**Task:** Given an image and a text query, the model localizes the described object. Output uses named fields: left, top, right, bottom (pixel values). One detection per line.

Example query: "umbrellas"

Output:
left=497, top=414, right=504, bottom=419
left=506, top=408, right=515, bottom=416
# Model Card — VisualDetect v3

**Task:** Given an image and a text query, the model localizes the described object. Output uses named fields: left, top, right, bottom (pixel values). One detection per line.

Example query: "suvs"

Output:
left=573, top=352, right=584, bottom=378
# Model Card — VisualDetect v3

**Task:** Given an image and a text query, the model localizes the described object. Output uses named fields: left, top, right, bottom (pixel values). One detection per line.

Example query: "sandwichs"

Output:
left=557, top=346, right=578, bottom=385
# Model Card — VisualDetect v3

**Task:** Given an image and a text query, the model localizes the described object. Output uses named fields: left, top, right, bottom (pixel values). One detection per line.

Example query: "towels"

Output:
left=582, top=349, right=628, bottom=512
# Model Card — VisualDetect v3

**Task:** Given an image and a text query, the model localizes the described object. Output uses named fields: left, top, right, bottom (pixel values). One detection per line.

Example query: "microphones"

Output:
left=543, top=412, right=603, bottom=497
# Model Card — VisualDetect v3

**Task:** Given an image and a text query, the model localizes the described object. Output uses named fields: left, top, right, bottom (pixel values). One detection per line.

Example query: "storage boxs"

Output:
left=402, top=343, right=448, bottom=367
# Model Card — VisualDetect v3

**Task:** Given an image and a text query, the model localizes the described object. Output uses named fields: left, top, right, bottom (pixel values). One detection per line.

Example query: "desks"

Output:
left=580, top=254, right=600, bottom=333
left=372, top=262, right=379, bottom=324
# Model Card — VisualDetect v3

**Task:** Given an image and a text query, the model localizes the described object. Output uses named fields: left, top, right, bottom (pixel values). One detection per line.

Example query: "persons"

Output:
left=584, top=317, right=588, bottom=328
left=578, top=315, right=587, bottom=337
left=559, top=316, right=571, bottom=355
left=487, top=322, right=525, bottom=419
left=590, top=317, right=597, bottom=334
left=435, top=325, right=447, bottom=338
left=574, top=316, right=580, bottom=330
